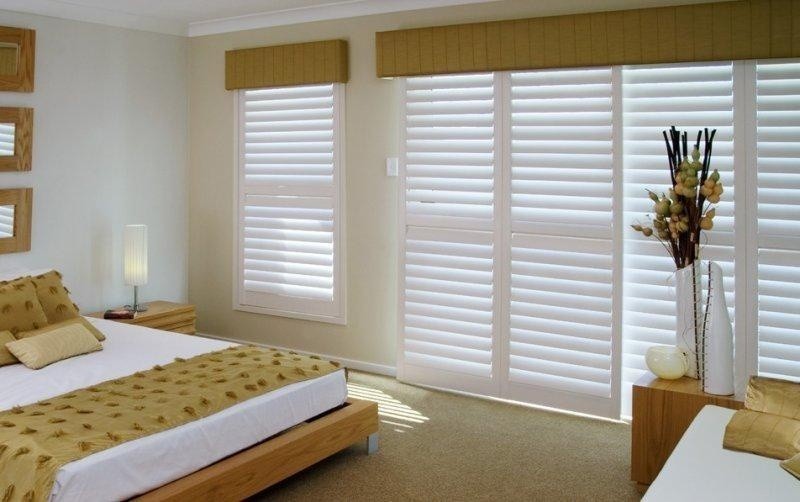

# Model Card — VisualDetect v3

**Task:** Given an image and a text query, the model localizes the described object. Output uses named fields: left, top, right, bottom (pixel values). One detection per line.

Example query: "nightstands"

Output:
left=85, top=300, right=197, bottom=336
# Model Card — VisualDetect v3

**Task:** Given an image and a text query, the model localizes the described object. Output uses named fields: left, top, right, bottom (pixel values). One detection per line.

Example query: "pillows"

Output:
left=0, top=269, right=106, bottom=368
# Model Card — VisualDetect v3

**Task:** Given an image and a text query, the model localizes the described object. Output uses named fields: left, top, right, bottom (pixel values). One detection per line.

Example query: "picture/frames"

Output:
left=0, top=187, right=33, bottom=255
left=0, top=106, right=34, bottom=172
left=1, top=24, right=36, bottom=94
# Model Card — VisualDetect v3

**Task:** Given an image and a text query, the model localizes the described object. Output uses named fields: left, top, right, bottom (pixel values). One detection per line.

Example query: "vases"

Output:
left=701, top=260, right=737, bottom=396
left=676, top=259, right=704, bottom=381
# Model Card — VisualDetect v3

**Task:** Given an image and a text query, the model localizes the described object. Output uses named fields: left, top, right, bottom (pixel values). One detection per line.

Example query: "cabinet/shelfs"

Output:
left=625, top=363, right=744, bottom=488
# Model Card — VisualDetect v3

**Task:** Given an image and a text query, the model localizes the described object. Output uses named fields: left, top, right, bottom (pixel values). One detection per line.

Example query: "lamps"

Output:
left=124, top=225, right=150, bottom=311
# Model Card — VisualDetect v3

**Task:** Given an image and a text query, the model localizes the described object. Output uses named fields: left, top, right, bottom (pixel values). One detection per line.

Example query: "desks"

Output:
left=638, top=405, right=800, bottom=502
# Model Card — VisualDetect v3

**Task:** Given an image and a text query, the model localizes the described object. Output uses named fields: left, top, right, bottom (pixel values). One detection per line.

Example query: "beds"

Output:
left=0, top=270, right=382, bottom=501
